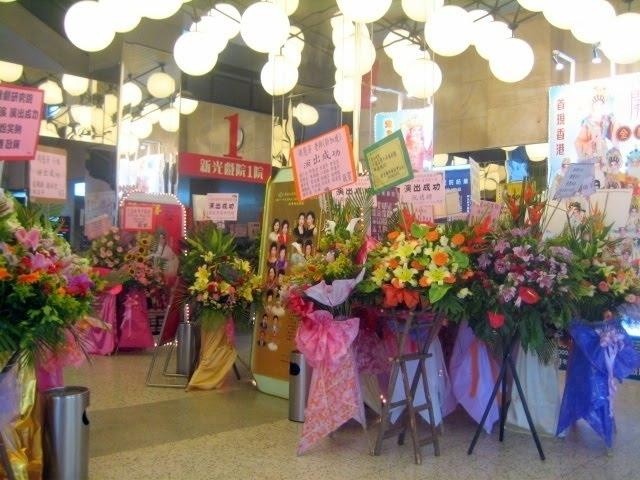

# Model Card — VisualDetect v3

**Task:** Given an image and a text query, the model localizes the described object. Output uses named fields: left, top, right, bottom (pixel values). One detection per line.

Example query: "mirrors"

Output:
left=433, top=142, right=547, bottom=204
left=114, top=41, right=184, bottom=204
left=269, top=0, right=435, bottom=175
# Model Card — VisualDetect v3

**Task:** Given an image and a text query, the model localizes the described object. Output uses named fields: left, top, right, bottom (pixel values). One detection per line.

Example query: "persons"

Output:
left=254, top=204, right=318, bottom=348
left=153, top=229, right=179, bottom=278
left=404, top=125, right=435, bottom=173
left=570, top=91, right=622, bottom=163
left=550, top=147, right=640, bottom=267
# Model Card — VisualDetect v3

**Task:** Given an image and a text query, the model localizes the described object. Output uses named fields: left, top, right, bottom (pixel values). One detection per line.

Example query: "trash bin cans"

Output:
left=40, top=385, right=90, bottom=480
left=176, top=302, right=200, bottom=375
left=288, top=350, right=313, bottom=422
